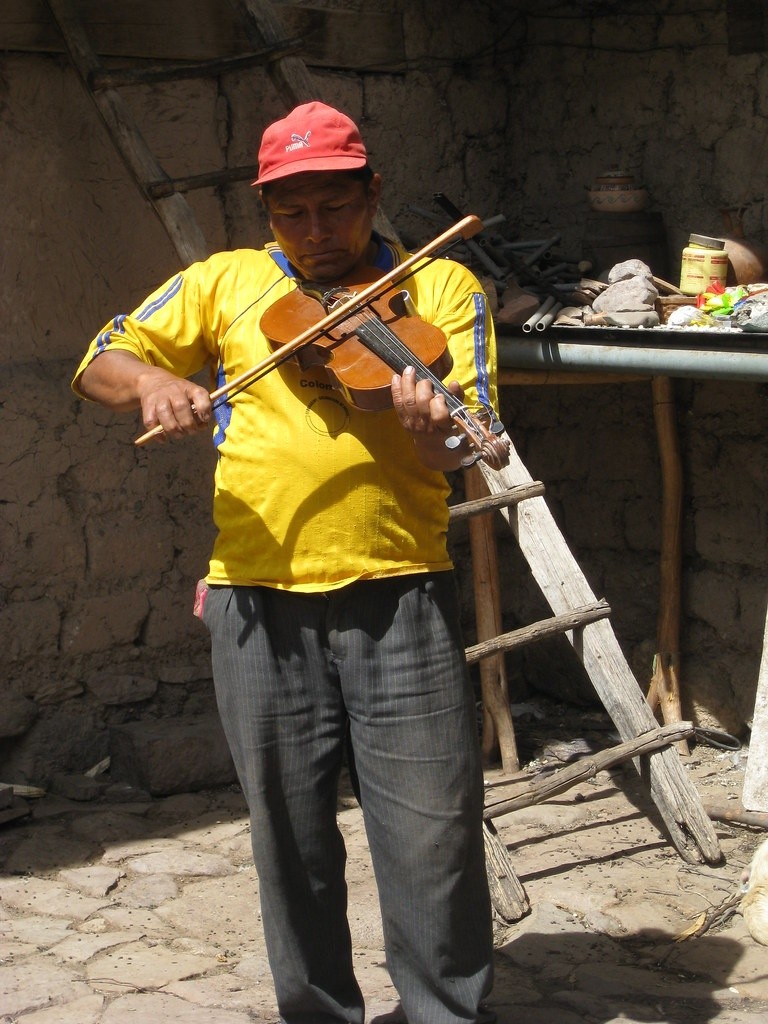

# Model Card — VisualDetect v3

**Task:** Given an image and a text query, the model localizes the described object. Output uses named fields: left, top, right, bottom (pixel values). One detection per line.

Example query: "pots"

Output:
left=582, top=174, right=651, bottom=213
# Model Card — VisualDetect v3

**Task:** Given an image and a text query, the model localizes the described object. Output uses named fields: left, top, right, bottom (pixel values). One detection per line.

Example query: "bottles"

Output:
left=680, top=233, right=728, bottom=294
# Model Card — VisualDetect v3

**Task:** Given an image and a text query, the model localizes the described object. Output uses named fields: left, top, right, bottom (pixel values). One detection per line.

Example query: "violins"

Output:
left=259, top=262, right=514, bottom=473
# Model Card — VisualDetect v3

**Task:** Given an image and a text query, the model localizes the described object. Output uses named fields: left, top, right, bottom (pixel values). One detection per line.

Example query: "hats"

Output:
left=249, top=102, right=369, bottom=188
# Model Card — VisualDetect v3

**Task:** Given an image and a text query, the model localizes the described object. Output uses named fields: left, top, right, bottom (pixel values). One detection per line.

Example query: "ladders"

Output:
left=50, top=0, right=725, bottom=922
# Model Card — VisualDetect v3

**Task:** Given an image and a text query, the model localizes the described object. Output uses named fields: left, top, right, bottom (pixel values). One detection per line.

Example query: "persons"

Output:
left=68, top=102, right=499, bottom=1023
